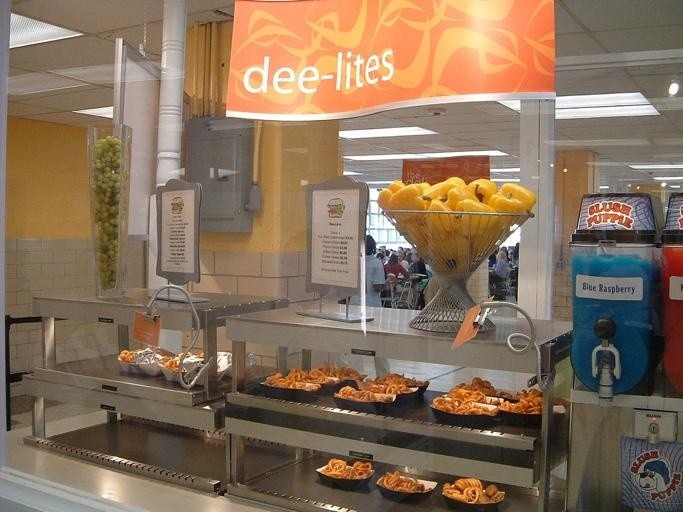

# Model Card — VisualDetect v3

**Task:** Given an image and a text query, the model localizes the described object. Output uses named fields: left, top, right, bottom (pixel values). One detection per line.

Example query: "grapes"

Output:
left=89, top=135, right=123, bottom=290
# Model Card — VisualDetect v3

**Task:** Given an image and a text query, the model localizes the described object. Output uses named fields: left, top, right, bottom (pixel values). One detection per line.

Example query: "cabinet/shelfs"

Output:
left=22, top=296, right=290, bottom=498
left=224, top=298, right=573, bottom=511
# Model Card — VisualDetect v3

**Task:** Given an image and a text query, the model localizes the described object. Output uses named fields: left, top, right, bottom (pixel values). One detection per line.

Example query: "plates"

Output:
left=431, top=376, right=569, bottom=430
left=256, top=362, right=430, bottom=412
left=441, top=482, right=506, bottom=511
left=373, top=475, right=439, bottom=503
left=314, top=462, right=377, bottom=487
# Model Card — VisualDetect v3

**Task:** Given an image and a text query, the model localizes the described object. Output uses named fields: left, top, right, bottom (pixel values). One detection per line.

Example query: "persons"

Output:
left=348, top=235, right=519, bottom=309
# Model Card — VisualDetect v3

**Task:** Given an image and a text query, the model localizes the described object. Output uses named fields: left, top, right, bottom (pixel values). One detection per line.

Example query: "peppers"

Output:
left=376, top=176, right=537, bottom=279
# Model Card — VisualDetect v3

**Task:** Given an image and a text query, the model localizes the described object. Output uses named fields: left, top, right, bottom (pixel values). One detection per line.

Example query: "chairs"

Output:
left=382, top=263, right=519, bottom=310
